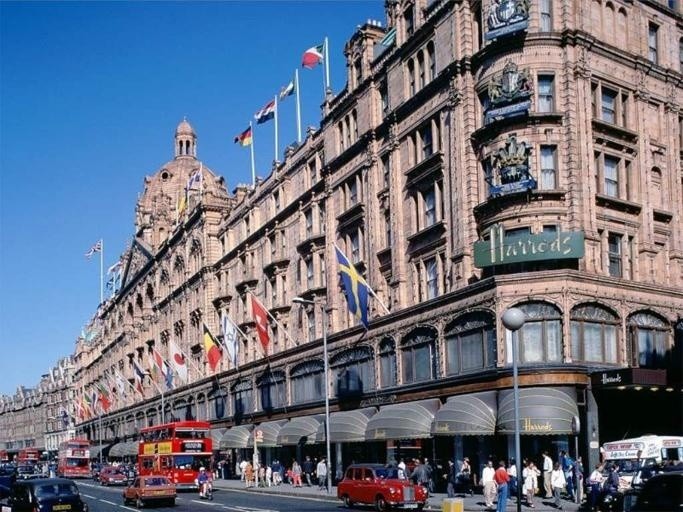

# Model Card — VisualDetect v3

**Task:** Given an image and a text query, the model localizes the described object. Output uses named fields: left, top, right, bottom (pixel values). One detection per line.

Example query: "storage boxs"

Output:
left=442, top=497, right=464, bottom=512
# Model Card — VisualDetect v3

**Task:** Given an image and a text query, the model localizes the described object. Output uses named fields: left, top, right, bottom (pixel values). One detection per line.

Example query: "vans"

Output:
left=586, top=435, right=683, bottom=495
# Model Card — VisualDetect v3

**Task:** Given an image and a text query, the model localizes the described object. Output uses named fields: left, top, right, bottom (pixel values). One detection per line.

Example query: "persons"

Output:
left=199, top=457, right=327, bottom=501
left=92, top=454, right=140, bottom=488
left=384, top=451, right=620, bottom=512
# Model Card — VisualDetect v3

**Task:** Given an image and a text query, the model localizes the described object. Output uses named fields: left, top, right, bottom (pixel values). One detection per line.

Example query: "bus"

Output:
left=138, top=421, right=213, bottom=489
left=57, top=439, right=92, bottom=479
left=18, top=448, right=40, bottom=463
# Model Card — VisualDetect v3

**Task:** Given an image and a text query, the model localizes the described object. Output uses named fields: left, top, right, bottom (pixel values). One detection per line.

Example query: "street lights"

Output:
left=87, top=403, right=103, bottom=463
left=503, top=309, right=526, bottom=511
left=292, top=296, right=331, bottom=493
left=142, top=370, right=165, bottom=425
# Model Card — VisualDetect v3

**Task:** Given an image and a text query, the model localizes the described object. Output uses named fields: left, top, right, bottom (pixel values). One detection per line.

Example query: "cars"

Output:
left=2, top=460, right=89, bottom=512
left=338, top=463, right=428, bottom=512
left=121, top=475, right=177, bottom=509
left=92, top=463, right=128, bottom=486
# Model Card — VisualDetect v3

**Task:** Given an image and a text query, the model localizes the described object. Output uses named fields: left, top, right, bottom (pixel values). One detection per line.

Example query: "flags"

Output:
left=85, top=241, right=101, bottom=260
left=280, top=80, right=295, bottom=100
left=335, top=245, right=367, bottom=331
left=234, top=127, right=252, bottom=147
left=188, top=170, right=200, bottom=188
left=252, top=296, right=270, bottom=355
left=60, top=314, right=238, bottom=427
left=302, top=44, right=324, bottom=69
left=255, top=100, right=275, bottom=125
left=179, top=192, right=187, bottom=216
